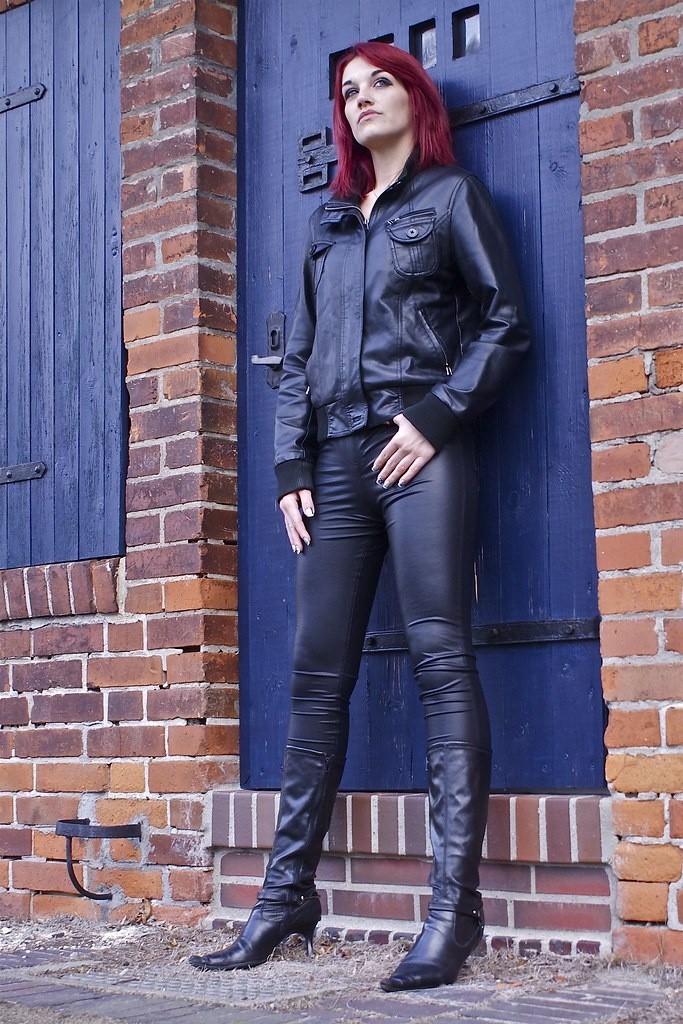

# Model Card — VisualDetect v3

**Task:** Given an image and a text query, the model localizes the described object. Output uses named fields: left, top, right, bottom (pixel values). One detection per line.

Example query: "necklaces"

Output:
left=372, top=169, right=403, bottom=199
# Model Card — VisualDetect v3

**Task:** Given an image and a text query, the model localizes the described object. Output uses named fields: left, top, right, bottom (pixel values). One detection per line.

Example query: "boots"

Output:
left=383, top=743, right=486, bottom=990
left=187, top=746, right=347, bottom=971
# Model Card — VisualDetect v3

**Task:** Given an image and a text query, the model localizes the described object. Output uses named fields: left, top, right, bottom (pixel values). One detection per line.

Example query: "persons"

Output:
left=189, top=44, right=532, bottom=991
